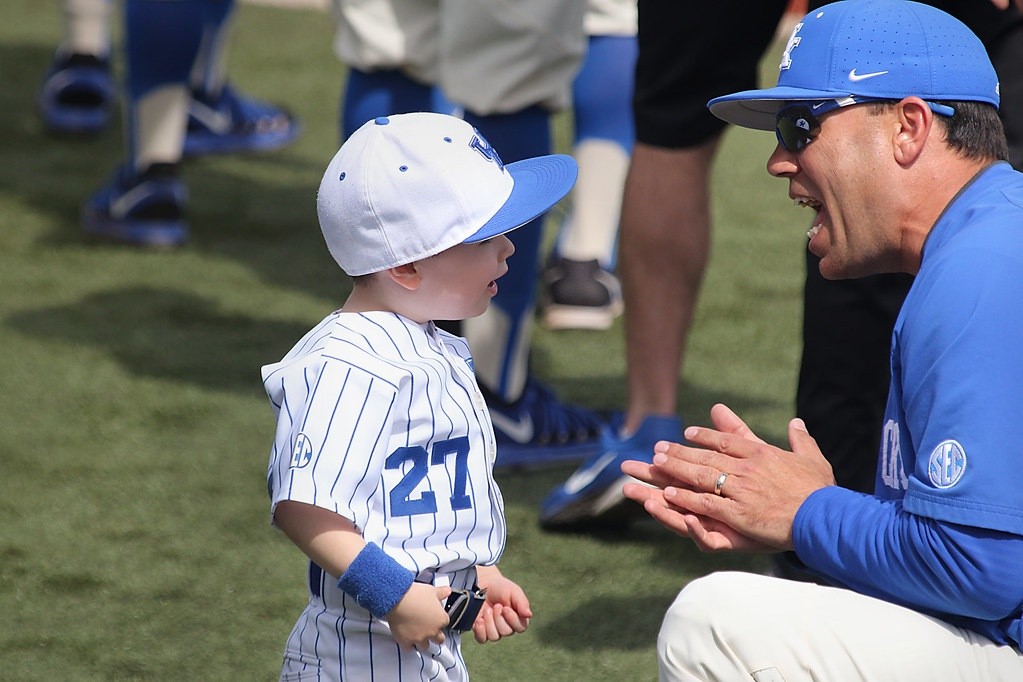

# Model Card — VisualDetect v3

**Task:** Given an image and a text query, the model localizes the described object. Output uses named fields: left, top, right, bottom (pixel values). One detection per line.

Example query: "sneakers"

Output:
left=536, top=412, right=689, bottom=529
left=473, top=373, right=625, bottom=466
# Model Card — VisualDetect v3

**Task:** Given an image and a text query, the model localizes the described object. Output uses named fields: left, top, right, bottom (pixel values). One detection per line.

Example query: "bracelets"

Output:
left=336, top=542, right=416, bottom=619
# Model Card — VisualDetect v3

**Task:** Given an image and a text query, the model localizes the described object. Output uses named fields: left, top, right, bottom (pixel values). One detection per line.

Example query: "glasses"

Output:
left=774, top=96, right=955, bottom=152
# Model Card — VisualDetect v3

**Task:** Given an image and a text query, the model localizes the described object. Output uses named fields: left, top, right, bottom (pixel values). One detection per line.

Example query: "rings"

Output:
left=714, top=472, right=727, bottom=495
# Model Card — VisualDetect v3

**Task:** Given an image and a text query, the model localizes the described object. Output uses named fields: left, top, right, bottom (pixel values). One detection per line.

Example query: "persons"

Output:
left=328, top=0, right=1023, bottom=532
left=259, top=112, right=578, bottom=682
left=39, top=0, right=302, bottom=244
left=622, top=0, right=1023, bottom=682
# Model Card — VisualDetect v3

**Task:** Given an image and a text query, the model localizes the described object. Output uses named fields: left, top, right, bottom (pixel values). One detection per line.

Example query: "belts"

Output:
left=309, top=559, right=488, bottom=631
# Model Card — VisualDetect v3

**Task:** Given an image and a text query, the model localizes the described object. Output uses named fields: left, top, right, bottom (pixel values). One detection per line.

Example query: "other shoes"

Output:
left=38, top=52, right=115, bottom=136
left=535, top=248, right=625, bottom=329
left=79, top=160, right=186, bottom=244
left=181, top=82, right=297, bottom=154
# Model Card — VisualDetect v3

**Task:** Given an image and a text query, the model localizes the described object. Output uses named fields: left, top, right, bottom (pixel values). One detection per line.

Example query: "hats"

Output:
left=706, top=0, right=1000, bottom=131
left=317, top=112, right=579, bottom=276
